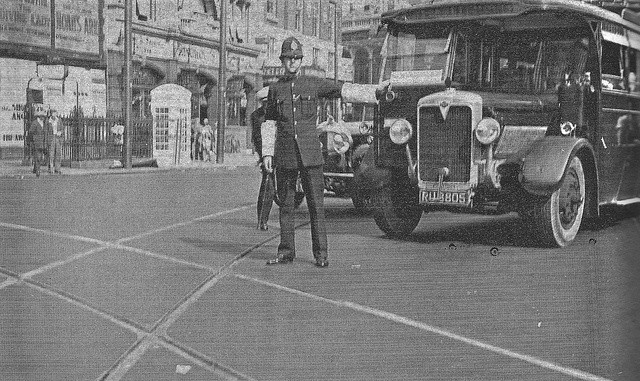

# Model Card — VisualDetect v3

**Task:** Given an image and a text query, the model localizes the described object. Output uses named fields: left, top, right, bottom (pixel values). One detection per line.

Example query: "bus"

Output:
left=370, top=0, right=640, bottom=247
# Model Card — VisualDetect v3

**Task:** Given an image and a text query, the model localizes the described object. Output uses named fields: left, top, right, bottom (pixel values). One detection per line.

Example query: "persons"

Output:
left=191, top=118, right=201, bottom=163
left=198, top=118, right=213, bottom=163
left=261, top=36, right=389, bottom=267
left=28, top=110, right=45, bottom=178
left=250, top=86, right=279, bottom=231
left=44, top=108, right=65, bottom=174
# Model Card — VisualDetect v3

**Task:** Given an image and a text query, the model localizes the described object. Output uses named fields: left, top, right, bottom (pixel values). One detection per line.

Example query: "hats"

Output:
left=257, top=87, right=269, bottom=101
left=279, top=37, right=304, bottom=59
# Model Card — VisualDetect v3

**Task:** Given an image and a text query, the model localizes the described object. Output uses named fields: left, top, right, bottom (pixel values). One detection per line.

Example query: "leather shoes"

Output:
left=316, top=259, right=328, bottom=267
left=267, top=256, right=294, bottom=264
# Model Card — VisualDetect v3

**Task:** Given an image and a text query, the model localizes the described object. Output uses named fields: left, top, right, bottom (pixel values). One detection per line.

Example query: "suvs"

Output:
left=275, top=96, right=382, bottom=208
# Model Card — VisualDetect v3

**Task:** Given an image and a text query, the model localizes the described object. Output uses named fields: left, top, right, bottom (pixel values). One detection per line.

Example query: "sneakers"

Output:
left=259, top=223, right=268, bottom=231
